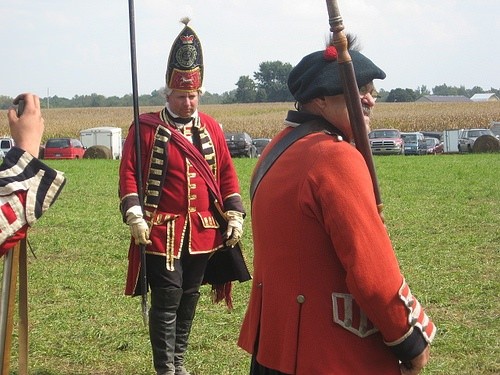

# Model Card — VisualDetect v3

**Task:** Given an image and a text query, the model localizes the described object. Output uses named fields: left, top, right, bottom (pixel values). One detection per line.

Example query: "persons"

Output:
left=118, top=89, right=251, bottom=375
left=235, top=49, right=438, bottom=375
left=0, top=93, right=67, bottom=259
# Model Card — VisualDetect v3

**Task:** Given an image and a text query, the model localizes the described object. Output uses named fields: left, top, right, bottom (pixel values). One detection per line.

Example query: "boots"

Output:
left=174, top=291, right=201, bottom=375
left=148, top=288, right=184, bottom=375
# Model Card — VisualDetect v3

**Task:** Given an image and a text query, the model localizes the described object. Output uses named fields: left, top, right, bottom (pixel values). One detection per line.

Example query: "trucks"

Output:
left=79, top=126, right=126, bottom=160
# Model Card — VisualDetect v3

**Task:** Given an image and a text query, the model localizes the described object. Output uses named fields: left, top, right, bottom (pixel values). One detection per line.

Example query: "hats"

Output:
left=287, top=48, right=387, bottom=104
left=165, top=26, right=204, bottom=92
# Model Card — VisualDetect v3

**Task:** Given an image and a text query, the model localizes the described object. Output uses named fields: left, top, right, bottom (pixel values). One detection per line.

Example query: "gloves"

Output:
left=125, top=206, right=154, bottom=247
left=223, top=211, right=244, bottom=249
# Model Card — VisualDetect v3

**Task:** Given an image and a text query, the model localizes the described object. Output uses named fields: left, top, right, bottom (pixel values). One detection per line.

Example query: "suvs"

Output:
left=457, top=128, right=500, bottom=154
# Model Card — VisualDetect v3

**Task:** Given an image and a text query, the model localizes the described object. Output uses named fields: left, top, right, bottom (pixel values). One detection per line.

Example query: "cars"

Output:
left=0, top=136, right=16, bottom=159
left=223, top=131, right=259, bottom=159
left=368, top=128, right=406, bottom=156
left=425, top=137, right=445, bottom=155
left=43, top=137, right=87, bottom=160
left=400, top=131, right=428, bottom=155
left=252, top=138, right=274, bottom=157
